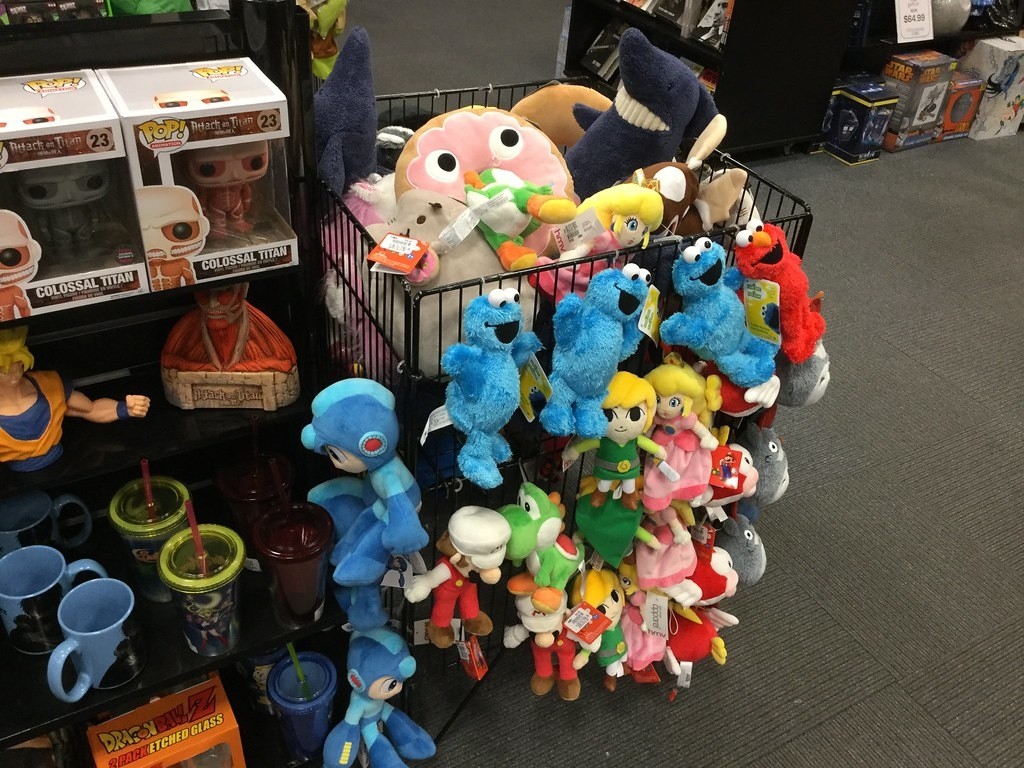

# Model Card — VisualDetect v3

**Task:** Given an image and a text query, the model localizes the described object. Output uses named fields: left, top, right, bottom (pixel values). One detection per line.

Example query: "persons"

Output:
left=2, top=324, right=152, bottom=503
left=692, top=2, right=727, bottom=48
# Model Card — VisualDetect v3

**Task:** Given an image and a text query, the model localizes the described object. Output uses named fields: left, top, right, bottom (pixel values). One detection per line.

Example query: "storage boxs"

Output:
left=1, top=69, right=151, bottom=321
left=820, top=33, right=1024, bottom=165
left=98, top=57, right=299, bottom=294
left=88, top=675, right=246, bottom=768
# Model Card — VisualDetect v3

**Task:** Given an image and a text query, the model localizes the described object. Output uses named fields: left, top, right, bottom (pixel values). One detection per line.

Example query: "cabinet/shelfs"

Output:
left=561, top=0, right=833, bottom=168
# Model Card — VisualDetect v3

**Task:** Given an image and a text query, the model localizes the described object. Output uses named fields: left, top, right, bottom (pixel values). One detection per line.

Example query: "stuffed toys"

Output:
left=215, top=28, right=835, bottom=768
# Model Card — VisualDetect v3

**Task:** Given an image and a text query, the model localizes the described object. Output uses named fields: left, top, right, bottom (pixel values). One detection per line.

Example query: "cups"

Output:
left=234, top=642, right=289, bottom=715
left=266, top=651, right=337, bottom=761
left=108, top=476, right=193, bottom=602
left=214, top=449, right=291, bottom=558
left=253, top=501, right=335, bottom=629
left=0, top=545, right=109, bottom=656
left=157, top=523, right=247, bottom=657
left=0, top=489, right=92, bottom=565
left=46, top=578, right=148, bottom=703
